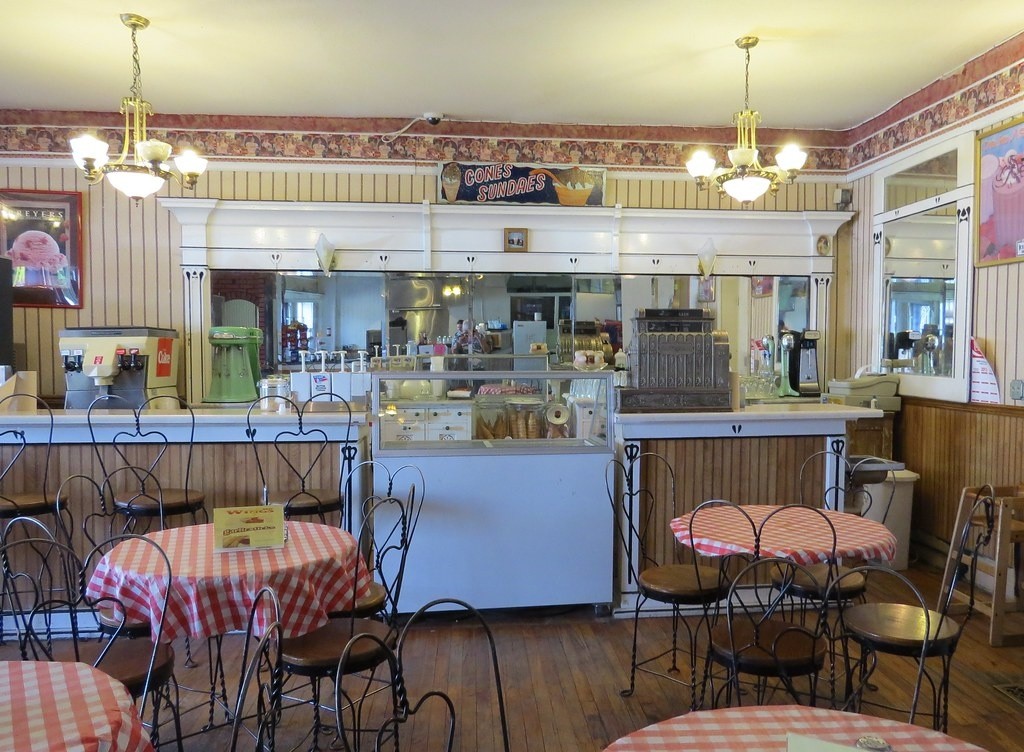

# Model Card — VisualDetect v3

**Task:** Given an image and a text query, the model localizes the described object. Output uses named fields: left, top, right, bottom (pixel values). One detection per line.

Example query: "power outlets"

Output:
left=1009, top=379, right=1024, bottom=401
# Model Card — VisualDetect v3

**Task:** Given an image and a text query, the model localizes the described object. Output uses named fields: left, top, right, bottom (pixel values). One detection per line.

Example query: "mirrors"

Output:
left=870, top=129, right=977, bottom=404
left=776, top=277, right=810, bottom=364
left=211, top=269, right=813, bottom=398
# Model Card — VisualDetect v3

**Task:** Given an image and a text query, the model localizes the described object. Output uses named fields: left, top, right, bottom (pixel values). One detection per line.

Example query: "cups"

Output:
left=259, top=379, right=285, bottom=412
left=326, top=327, right=331, bottom=336
left=534, top=312, right=543, bottom=321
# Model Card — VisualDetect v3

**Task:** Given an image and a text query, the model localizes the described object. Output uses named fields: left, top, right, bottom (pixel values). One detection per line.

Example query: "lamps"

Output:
left=689, top=36, right=809, bottom=206
left=68, top=12, right=210, bottom=209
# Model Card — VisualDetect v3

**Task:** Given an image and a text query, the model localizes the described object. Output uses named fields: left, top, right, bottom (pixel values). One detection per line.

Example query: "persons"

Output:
left=452, top=320, right=482, bottom=370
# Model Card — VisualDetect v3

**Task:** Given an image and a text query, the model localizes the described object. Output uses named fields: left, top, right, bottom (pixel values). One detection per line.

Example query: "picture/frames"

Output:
left=0, top=188, right=84, bottom=308
left=697, top=276, right=716, bottom=302
left=504, top=228, right=529, bottom=253
left=750, top=276, right=773, bottom=298
left=974, top=116, right=1024, bottom=269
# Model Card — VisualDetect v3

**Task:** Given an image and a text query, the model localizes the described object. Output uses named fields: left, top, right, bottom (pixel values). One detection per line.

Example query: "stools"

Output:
left=936, top=483, right=1024, bottom=646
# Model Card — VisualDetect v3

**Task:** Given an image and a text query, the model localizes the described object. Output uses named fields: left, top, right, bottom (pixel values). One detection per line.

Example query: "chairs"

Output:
left=334, top=597, right=511, bottom=752
left=317, top=460, right=426, bottom=718
left=85, top=394, right=210, bottom=534
left=272, top=483, right=417, bottom=752
left=831, top=482, right=996, bottom=735
left=0, top=534, right=173, bottom=721
left=231, top=586, right=284, bottom=752
left=55, top=465, right=231, bottom=752
left=767, top=439, right=897, bottom=709
left=688, top=497, right=837, bottom=714
left=246, top=392, right=352, bottom=531
left=608, top=445, right=735, bottom=713
left=0, top=392, right=79, bottom=659
left=0, top=515, right=184, bottom=752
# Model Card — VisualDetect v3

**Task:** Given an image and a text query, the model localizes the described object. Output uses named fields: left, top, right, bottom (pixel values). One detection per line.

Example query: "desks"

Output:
left=0, top=660, right=132, bottom=752
left=849, top=411, right=897, bottom=460
left=603, top=705, right=991, bottom=752
left=668, top=505, right=898, bottom=708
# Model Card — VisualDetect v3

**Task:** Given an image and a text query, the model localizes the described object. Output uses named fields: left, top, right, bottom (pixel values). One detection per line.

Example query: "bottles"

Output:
left=871, top=395, right=878, bottom=409
left=423, top=332, right=427, bottom=344
left=407, top=341, right=417, bottom=354
left=615, top=303, right=622, bottom=322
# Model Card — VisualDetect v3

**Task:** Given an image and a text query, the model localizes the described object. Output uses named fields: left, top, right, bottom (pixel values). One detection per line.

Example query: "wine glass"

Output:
left=740, top=376, right=779, bottom=397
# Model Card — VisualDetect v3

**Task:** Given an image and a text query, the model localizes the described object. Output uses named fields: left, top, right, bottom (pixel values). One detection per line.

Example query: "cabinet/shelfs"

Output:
left=370, top=369, right=617, bottom=617
left=381, top=409, right=477, bottom=448
left=567, top=397, right=607, bottom=438
